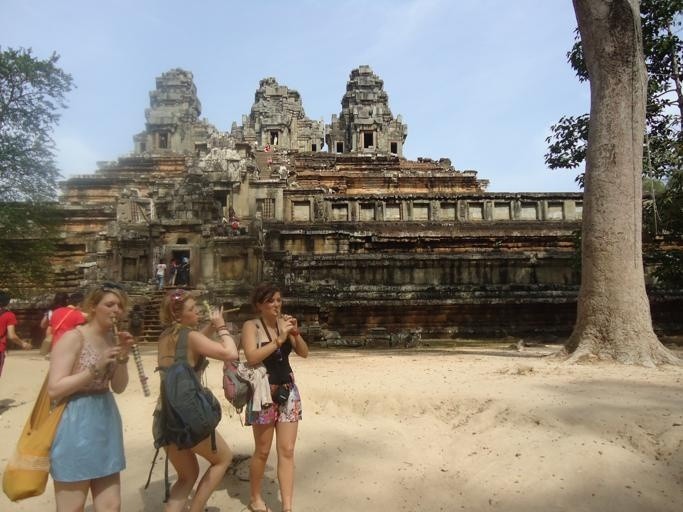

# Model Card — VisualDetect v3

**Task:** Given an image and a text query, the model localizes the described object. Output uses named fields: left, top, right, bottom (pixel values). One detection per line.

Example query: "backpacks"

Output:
left=162, top=329, right=221, bottom=450
left=223, top=320, right=260, bottom=408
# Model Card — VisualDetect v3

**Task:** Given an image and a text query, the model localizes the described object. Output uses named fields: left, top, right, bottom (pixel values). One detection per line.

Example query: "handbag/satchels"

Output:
left=40, top=335, right=52, bottom=355
left=1, top=327, right=85, bottom=502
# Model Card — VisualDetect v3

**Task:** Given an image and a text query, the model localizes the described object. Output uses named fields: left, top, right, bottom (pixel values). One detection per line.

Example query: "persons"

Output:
left=239, top=279, right=308, bottom=512
left=176, top=255, right=190, bottom=285
left=168, top=258, right=179, bottom=285
left=36, top=310, right=50, bottom=328
left=150, top=288, right=238, bottom=512
left=46, top=283, right=135, bottom=512
left=0, top=287, right=33, bottom=380
left=45, top=291, right=88, bottom=319
left=230, top=218, right=239, bottom=235
left=220, top=215, right=228, bottom=226
left=155, top=259, right=167, bottom=291
left=45, top=291, right=86, bottom=353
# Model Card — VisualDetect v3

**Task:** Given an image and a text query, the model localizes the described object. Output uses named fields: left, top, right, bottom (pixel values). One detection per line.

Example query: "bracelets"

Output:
left=289, top=329, right=300, bottom=336
left=87, top=364, right=99, bottom=377
left=273, top=337, right=280, bottom=348
left=117, top=358, right=128, bottom=363
left=216, top=333, right=231, bottom=338
left=215, top=325, right=229, bottom=332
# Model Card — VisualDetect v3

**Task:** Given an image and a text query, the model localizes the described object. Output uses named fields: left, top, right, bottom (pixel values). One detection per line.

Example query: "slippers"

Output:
left=248, top=502, right=273, bottom=512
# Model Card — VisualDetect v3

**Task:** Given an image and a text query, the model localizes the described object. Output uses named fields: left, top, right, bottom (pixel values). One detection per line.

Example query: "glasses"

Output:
left=171, top=288, right=184, bottom=311
left=95, top=282, right=123, bottom=290
